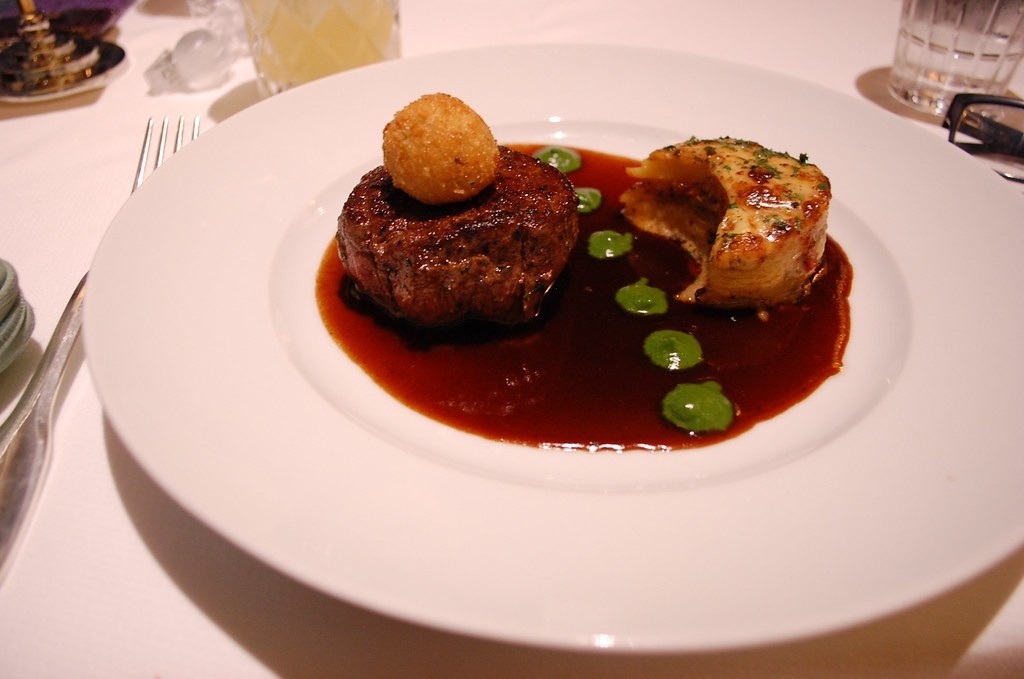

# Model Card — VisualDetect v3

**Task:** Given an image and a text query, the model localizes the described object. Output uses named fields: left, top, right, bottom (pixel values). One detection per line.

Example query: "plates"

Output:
left=79, top=41, right=1023, bottom=657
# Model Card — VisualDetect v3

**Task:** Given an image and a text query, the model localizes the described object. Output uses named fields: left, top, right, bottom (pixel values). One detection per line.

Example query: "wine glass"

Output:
left=0, top=0, right=126, bottom=101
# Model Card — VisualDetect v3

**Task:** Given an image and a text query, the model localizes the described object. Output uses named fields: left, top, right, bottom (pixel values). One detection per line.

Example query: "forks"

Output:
left=1, top=115, right=203, bottom=581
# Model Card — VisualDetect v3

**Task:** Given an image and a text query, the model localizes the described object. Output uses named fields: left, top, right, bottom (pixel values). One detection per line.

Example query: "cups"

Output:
left=887, top=0, right=1024, bottom=123
left=239, top=0, right=401, bottom=100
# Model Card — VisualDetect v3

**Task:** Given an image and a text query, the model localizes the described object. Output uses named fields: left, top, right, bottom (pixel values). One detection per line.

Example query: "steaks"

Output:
left=336, top=144, right=580, bottom=324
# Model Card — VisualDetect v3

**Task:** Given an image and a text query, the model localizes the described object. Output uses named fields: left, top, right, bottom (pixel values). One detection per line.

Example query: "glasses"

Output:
left=942, top=94, right=1024, bottom=184
